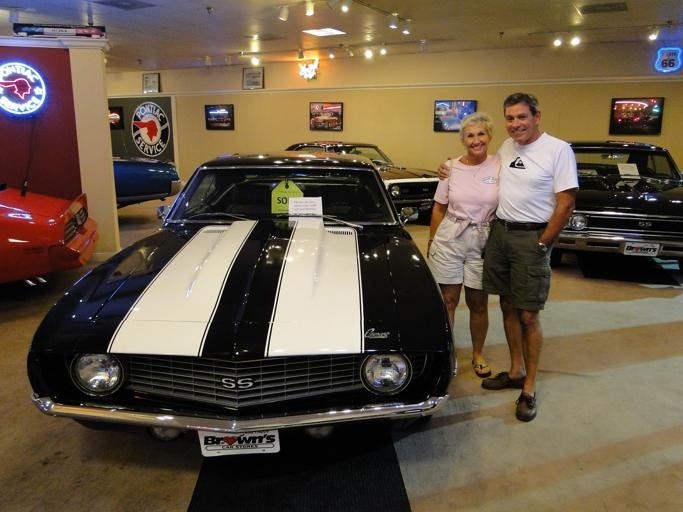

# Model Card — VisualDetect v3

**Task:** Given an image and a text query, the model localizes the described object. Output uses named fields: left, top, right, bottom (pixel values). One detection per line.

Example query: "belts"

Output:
left=496, top=218, right=547, bottom=230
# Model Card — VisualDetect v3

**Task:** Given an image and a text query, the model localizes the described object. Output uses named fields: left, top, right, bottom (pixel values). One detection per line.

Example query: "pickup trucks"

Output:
left=111, top=157, right=181, bottom=210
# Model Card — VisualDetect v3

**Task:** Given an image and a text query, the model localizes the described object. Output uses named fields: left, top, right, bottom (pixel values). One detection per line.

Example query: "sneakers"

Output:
left=482, top=373, right=526, bottom=390
left=516, top=391, right=536, bottom=421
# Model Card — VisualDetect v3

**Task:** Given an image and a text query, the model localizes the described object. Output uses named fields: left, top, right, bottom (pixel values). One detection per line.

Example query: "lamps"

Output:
left=272, top=0, right=413, bottom=36
left=647, top=23, right=660, bottom=43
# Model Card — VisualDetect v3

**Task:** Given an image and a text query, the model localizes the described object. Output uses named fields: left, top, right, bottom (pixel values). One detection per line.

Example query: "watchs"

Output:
left=536, top=240, right=548, bottom=252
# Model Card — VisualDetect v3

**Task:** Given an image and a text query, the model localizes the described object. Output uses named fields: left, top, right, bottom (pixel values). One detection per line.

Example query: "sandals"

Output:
left=472, top=359, right=491, bottom=377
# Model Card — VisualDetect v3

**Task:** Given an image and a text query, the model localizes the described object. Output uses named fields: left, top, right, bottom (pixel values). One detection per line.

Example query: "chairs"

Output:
left=224, top=180, right=271, bottom=219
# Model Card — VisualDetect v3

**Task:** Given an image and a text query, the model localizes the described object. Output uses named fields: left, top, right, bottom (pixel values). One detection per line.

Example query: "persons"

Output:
left=437, top=89, right=580, bottom=422
left=421, top=112, right=502, bottom=378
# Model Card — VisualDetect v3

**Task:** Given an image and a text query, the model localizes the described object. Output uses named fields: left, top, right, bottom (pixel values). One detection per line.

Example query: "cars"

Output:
left=434, top=104, right=474, bottom=132
left=310, top=114, right=340, bottom=130
left=284, top=141, right=439, bottom=227
left=26, top=151, right=457, bottom=457
left=548, top=141, right=682, bottom=281
left=0, top=113, right=98, bottom=285
